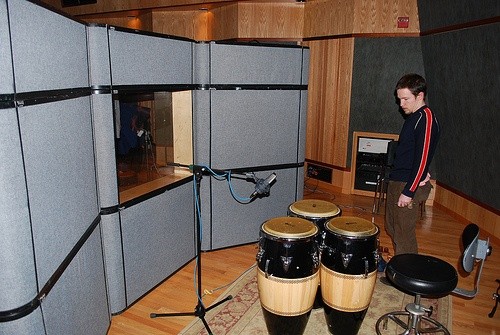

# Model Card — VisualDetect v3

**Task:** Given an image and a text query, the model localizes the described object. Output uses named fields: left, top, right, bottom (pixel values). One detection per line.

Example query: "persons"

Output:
left=380, top=73, right=441, bottom=287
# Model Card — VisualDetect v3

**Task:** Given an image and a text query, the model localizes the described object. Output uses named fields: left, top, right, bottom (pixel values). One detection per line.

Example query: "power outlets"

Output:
left=306, top=163, right=333, bottom=184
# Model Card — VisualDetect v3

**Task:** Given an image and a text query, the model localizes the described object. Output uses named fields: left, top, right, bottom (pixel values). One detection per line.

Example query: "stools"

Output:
left=375, top=223, right=492, bottom=335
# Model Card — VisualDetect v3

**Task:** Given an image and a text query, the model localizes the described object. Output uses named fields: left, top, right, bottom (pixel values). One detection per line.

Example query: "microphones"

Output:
left=250, top=172, right=277, bottom=200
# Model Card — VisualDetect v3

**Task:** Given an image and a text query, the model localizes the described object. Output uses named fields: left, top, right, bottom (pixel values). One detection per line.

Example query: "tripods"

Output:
left=150, top=162, right=232, bottom=335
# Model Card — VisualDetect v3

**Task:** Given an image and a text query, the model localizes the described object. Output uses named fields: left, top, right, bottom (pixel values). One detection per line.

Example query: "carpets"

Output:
left=177, top=256, right=452, bottom=335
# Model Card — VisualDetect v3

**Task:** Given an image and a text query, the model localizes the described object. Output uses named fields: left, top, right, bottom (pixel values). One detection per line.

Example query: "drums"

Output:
left=256, top=216, right=321, bottom=335
left=320, top=215, right=381, bottom=335
left=287, top=199, right=343, bottom=310
